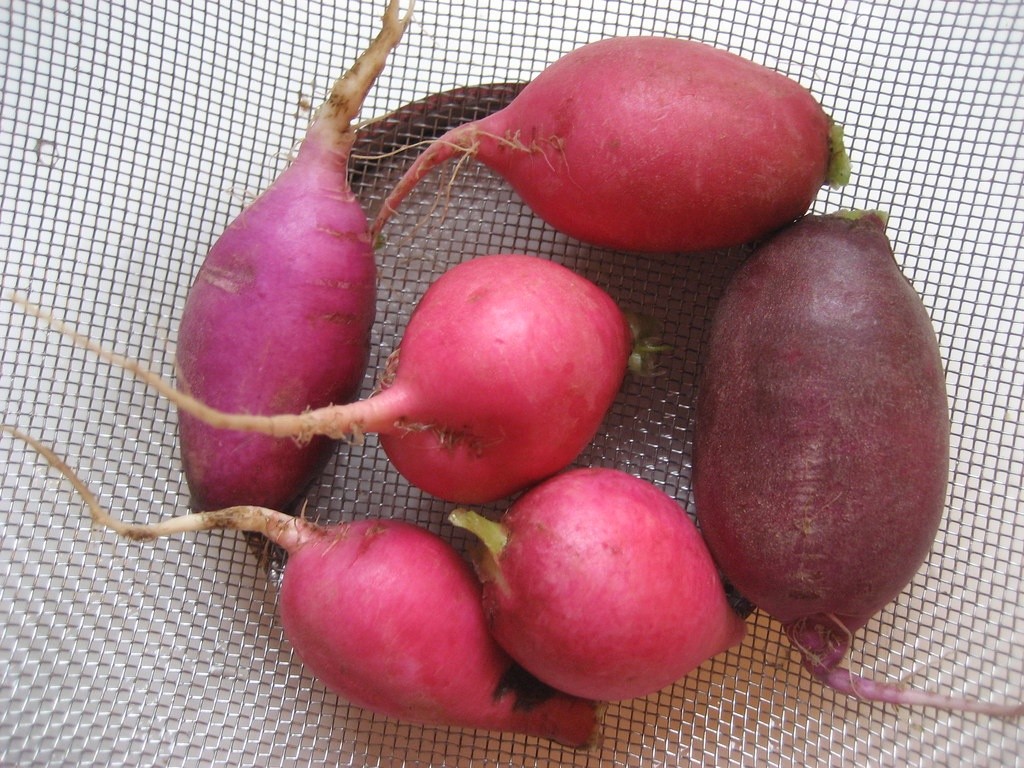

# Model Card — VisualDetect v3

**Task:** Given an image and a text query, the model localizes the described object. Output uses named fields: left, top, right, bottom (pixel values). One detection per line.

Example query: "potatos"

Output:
left=180, top=1, right=412, bottom=508
left=697, top=208, right=950, bottom=675
